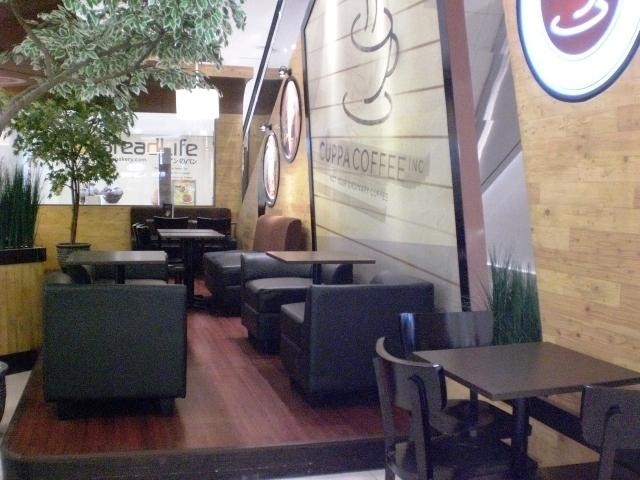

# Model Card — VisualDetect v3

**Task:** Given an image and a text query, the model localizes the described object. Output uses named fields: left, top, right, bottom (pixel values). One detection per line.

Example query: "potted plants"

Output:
left=3, top=87, right=131, bottom=273
